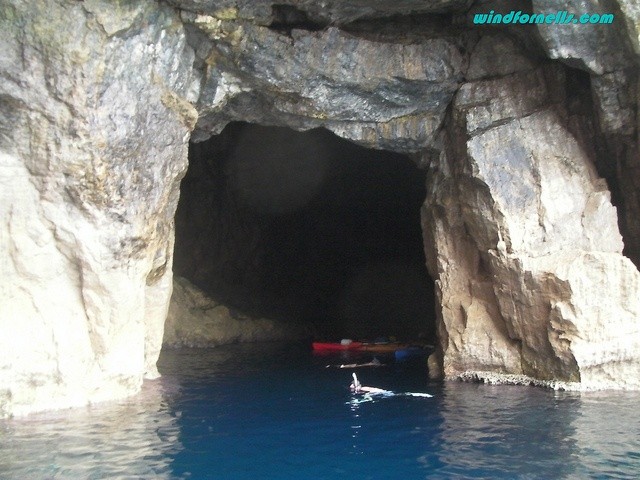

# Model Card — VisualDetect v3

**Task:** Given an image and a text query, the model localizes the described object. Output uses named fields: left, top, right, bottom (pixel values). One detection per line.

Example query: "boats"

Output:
left=312, top=341, right=363, bottom=352
left=359, top=341, right=410, bottom=352
left=395, top=345, right=434, bottom=357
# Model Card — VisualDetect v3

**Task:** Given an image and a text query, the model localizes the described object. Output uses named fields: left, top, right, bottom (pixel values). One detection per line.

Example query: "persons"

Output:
left=350, top=380, right=394, bottom=398
